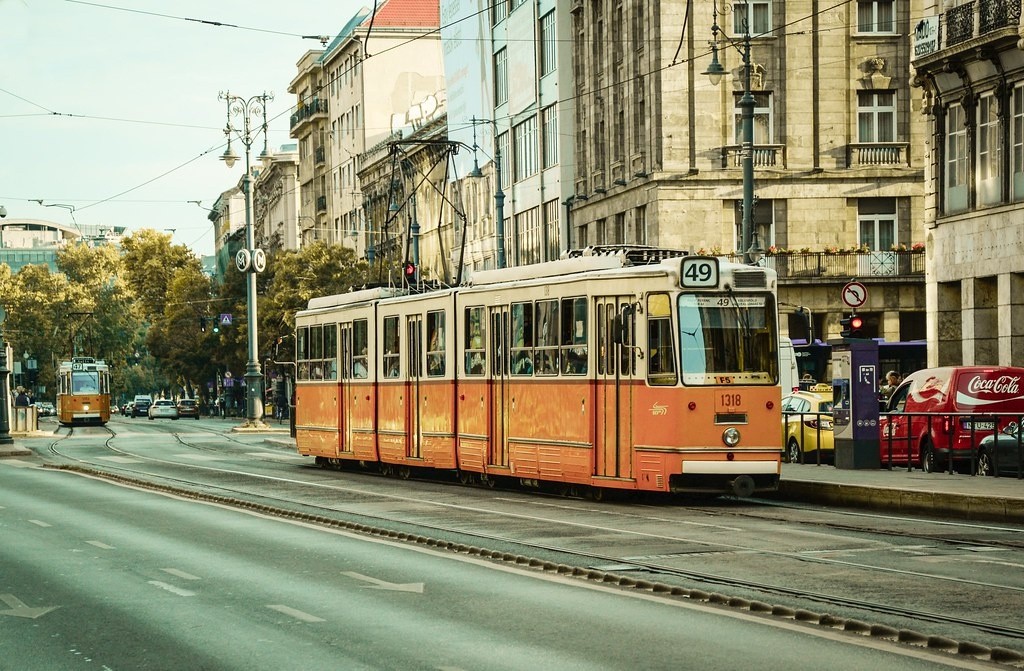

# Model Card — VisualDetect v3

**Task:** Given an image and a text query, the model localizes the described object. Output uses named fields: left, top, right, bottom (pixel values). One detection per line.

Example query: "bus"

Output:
left=272, top=243, right=812, bottom=499
left=55, top=356, right=112, bottom=427
left=791, top=337, right=927, bottom=391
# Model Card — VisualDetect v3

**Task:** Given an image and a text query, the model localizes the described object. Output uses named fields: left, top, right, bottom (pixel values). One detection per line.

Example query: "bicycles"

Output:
left=279, top=407, right=284, bottom=424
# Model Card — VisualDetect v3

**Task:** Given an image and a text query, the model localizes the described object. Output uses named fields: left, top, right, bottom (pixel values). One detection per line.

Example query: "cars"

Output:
left=979, top=415, right=1024, bottom=477
left=781, top=383, right=834, bottom=465
left=121, top=394, right=200, bottom=420
left=34, top=401, right=55, bottom=416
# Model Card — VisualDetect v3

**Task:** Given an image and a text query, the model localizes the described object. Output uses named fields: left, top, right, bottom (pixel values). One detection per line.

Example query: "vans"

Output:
left=778, top=336, right=799, bottom=399
left=879, top=366, right=1024, bottom=473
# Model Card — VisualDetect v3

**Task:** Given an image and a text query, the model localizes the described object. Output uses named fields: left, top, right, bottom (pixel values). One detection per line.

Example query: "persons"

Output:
left=80, top=383, right=94, bottom=393
left=208, top=390, right=289, bottom=420
left=878, top=371, right=909, bottom=401
left=14, top=386, right=35, bottom=408
left=300, top=338, right=589, bottom=376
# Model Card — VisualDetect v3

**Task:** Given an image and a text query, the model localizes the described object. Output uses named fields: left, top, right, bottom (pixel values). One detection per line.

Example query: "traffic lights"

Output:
left=406, top=261, right=415, bottom=276
left=840, top=317, right=851, bottom=338
left=851, top=316, right=863, bottom=338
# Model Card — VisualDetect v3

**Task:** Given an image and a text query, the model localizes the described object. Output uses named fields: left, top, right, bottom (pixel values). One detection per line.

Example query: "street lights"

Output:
left=464, top=115, right=507, bottom=271
left=220, top=86, right=290, bottom=433
left=699, top=2, right=756, bottom=266
